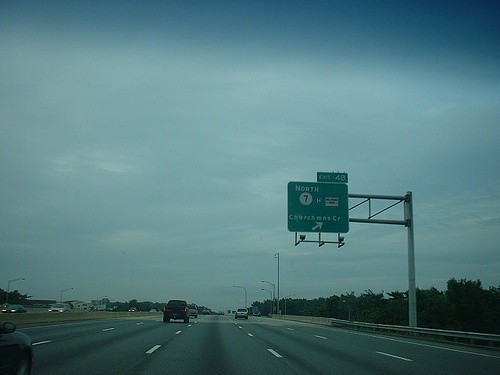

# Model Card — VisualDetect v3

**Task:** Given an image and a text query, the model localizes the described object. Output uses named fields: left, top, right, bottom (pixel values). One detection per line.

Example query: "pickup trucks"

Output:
left=163, top=300, right=198, bottom=324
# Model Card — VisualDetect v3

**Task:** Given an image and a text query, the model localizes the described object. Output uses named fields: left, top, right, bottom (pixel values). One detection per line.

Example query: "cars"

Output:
left=128, top=308, right=137, bottom=312
left=48, top=303, right=70, bottom=312
left=149, top=309, right=157, bottom=313
left=0, top=321, right=34, bottom=375
left=234, top=308, right=249, bottom=319
left=3, top=304, right=26, bottom=314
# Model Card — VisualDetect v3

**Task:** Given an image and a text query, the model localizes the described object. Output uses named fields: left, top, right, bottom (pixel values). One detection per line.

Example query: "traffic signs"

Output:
left=286, top=181, right=349, bottom=233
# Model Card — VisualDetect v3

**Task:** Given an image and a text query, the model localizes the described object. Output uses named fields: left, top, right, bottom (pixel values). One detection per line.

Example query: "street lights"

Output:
left=274, top=252, right=280, bottom=313
left=60, top=287, right=74, bottom=302
left=233, top=285, right=247, bottom=307
left=7, top=277, right=26, bottom=302
left=261, top=280, right=276, bottom=314
left=284, top=294, right=296, bottom=315
